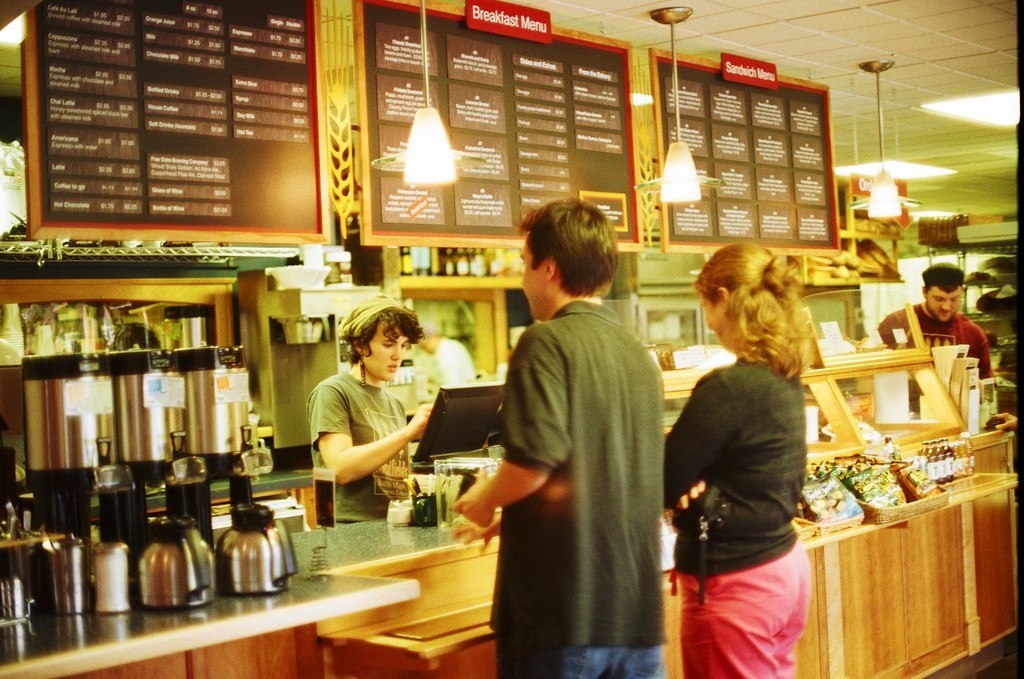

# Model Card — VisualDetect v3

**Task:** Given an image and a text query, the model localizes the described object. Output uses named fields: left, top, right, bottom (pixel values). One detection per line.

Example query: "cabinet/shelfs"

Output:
left=237, top=246, right=535, bottom=446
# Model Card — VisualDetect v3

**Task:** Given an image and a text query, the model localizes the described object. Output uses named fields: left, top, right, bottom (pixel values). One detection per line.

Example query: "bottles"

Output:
left=399, top=245, right=525, bottom=277
left=884, top=436, right=902, bottom=464
left=919, top=432, right=976, bottom=486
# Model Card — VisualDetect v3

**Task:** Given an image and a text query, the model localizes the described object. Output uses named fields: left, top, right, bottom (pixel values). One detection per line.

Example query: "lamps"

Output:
left=649, top=7, right=703, bottom=202
left=860, top=60, right=902, bottom=218
left=403, top=0, right=457, bottom=185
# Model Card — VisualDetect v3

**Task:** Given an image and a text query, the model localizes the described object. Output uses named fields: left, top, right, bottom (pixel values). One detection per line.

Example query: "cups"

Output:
left=0, top=302, right=26, bottom=366
left=412, top=493, right=446, bottom=527
left=32, top=323, right=55, bottom=356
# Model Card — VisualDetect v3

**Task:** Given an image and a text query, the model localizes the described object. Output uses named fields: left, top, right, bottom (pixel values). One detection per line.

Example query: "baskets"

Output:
left=857, top=482, right=950, bottom=525
left=795, top=524, right=817, bottom=542
left=918, top=214, right=1004, bottom=248
left=794, top=516, right=865, bottom=536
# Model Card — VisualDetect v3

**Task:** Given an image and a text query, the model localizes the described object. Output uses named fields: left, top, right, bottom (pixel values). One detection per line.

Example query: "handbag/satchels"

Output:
left=672, top=487, right=731, bottom=604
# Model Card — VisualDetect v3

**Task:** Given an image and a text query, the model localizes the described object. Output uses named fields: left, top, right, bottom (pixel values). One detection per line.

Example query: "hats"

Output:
left=922, top=262, right=965, bottom=286
left=336, top=293, right=401, bottom=338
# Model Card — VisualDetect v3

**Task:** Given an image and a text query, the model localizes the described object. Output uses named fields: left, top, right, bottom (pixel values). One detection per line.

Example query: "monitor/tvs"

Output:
left=412, top=381, right=506, bottom=461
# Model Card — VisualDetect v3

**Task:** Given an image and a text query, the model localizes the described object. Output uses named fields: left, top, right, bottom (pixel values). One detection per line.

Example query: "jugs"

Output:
left=135, top=505, right=299, bottom=611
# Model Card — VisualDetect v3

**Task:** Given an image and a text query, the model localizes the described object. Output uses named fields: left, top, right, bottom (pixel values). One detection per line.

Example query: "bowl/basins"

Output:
left=268, top=263, right=331, bottom=292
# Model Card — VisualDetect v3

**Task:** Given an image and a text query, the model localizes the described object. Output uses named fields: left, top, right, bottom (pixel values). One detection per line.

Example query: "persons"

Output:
left=664, top=243, right=818, bottom=678
left=409, top=317, right=476, bottom=394
left=452, top=199, right=666, bottom=678
left=985, top=413, right=1018, bottom=430
left=304, top=294, right=435, bottom=521
left=494, top=324, right=530, bottom=383
left=870, top=262, right=996, bottom=412
